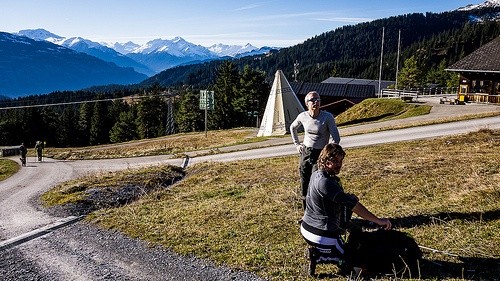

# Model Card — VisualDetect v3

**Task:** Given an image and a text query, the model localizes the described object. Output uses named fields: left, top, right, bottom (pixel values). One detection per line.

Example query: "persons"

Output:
left=290, top=91, right=340, bottom=212
left=20, top=143, right=27, bottom=164
left=35, top=141, right=44, bottom=161
left=300, top=144, right=392, bottom=276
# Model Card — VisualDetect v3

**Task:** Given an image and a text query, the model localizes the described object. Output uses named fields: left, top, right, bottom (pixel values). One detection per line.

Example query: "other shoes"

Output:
left=307, top=247, right=317, bottom=276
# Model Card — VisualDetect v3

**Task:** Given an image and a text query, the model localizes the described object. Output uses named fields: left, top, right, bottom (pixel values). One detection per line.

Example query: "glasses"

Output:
left=308, top=97, right=321, bottom=102
